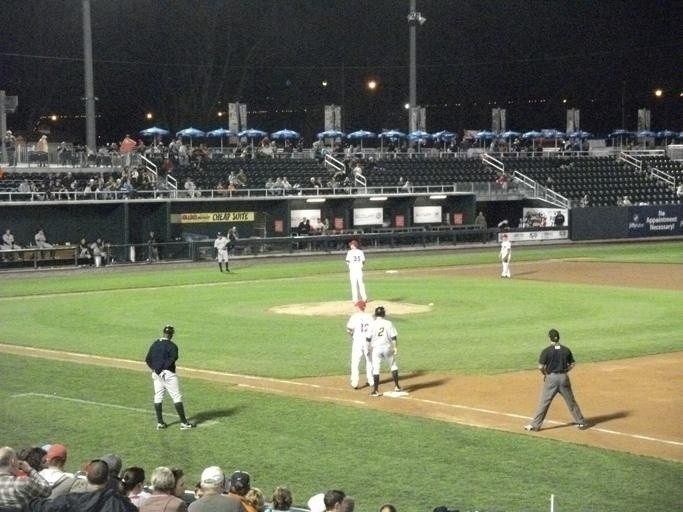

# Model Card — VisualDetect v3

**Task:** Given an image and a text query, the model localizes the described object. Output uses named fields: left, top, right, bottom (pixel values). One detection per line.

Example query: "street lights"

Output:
left=407, top=9, right=427, bottom=137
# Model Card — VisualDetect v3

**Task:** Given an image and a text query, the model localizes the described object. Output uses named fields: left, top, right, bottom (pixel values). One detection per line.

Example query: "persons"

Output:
left=524, top=328, right=588, bottom=435
left=345, top=239, right=367, bottom=302
left=498, top=234, right=511, bottom=277
left=144, top=324, right=193, bottom=431
left=365, top=306, right=402, bottom=392
left=345, top=302, right=369, bottom=389
left=2, top=129, right=683, bottom=267
left=186, top=467, right=448, bottom=511
left=214, top=232, right=229, bottom=272
left=1, top=444, right=184, bottom=512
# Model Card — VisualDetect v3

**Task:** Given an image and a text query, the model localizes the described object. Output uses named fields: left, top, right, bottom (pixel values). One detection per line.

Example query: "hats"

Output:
left=549, top=329, right=560, bottom=343
left=163, top=325, right=173, bottom=335
left=229, top=470, right=250, bottom=489
left=100, top=453, right=123, bottom=476
left=349, top=239, right=360, bottom=248
left=40, top=444, right=68, bottom=463
left=354, top=301, right=367, bottom=311
left=306, top=492, right=328, bottom=512
left=199, top=466, right=226, bottom=489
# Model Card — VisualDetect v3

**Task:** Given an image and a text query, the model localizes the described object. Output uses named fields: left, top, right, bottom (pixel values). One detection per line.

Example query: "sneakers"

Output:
left=578, top=418, right=591, bottom=430
left=523, top=424, right=541, bottom=431
left=156, top=422, right=167, bottom=431
left=369, top=390, right=380, bottom=397
left=395, top=385, right=404, bottom=392
left=180, top=422, right=195, bottom=429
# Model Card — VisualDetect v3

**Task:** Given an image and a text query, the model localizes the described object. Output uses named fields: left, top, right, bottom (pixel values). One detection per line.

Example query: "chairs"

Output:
left=0, top=141, right=682, bottom=261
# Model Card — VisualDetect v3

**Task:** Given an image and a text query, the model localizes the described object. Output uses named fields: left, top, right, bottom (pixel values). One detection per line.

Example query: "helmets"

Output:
left=375, top=306, right=386, bottom=315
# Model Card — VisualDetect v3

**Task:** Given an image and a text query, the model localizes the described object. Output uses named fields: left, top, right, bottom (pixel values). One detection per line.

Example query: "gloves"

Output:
left=158, top=372, right=166, bottom=382
left=151, top=372, right=158, bottom=380
left=393, top=347, right=398, bottom=356
left=366, top=347, right=371, bottom=355
